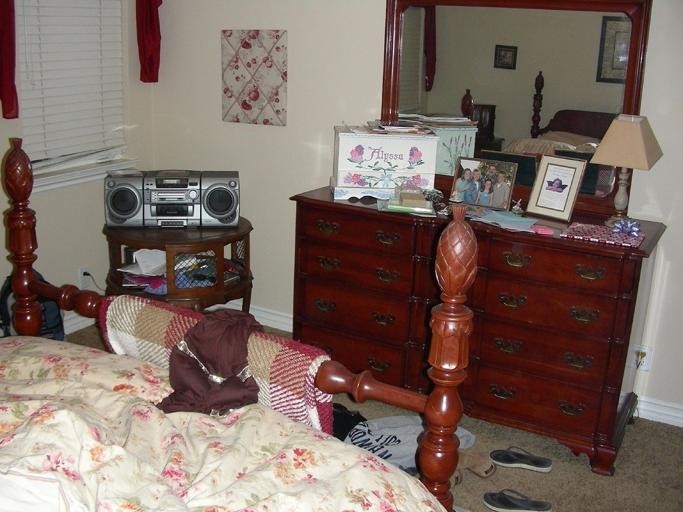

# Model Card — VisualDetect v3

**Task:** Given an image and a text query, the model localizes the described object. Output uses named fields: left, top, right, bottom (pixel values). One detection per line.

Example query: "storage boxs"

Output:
left=328, top=123, right=439, bottom=201
left=426, top=125, right=478, bottom=177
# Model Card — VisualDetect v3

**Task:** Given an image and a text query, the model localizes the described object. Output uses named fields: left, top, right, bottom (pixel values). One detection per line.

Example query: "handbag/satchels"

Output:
left=0, top=267, right=66, bottom=342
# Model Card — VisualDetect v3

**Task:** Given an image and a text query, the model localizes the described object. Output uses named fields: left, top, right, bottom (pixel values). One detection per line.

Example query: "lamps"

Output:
left=586, top=114, right=663, bottom=230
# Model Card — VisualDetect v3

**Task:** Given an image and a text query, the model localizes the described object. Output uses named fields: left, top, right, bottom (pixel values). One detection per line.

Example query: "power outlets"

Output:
left=77, top=262, right=93, bottom=289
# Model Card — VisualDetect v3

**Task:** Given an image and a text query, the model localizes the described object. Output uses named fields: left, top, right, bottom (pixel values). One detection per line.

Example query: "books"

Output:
left=376, top=186, right=436, bottom=215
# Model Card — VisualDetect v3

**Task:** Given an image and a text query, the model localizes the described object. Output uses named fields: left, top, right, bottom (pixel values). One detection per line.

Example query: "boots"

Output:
left=457, top=447, right=498, bottom=480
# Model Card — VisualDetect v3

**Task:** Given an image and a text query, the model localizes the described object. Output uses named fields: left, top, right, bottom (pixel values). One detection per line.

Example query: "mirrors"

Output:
left=380, top=0, right=654, bottom=219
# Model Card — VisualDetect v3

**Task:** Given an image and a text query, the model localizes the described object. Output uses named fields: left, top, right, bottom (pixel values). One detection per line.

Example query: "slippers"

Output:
left=482, top=488, right=553, bottom=512
left=487, top=445, right=555, bottom=474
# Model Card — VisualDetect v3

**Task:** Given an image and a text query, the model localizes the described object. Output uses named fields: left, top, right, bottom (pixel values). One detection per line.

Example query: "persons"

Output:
left=546, top=178, right=562, bottom=192
left=454, top=164, right=510, bottom=209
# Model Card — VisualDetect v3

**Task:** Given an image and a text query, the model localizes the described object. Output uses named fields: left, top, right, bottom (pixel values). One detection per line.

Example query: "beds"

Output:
left=0, top=134, right=484, bottom=512
left=498, top=69, right=617, bottom=188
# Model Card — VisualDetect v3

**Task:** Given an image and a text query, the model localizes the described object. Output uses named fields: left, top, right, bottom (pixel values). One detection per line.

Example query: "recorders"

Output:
left=104, top=170, right=240, bottom=228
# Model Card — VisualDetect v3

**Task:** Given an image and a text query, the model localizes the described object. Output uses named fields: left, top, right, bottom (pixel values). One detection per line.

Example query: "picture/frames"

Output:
left=595, top=13, right=632, bottom=86
left=524, top=151, right=588, bottom=224
left=492, top=42, right=518, bottom=70
left=448, top=155, right=519, bottom=212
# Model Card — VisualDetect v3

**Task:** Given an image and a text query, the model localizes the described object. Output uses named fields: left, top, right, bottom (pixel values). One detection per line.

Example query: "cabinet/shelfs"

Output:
left=100, top=216, right=256, bottom=319
left=446, top=210, right=667, bottom=476
left=284, top=184, right=447, bottom=399
